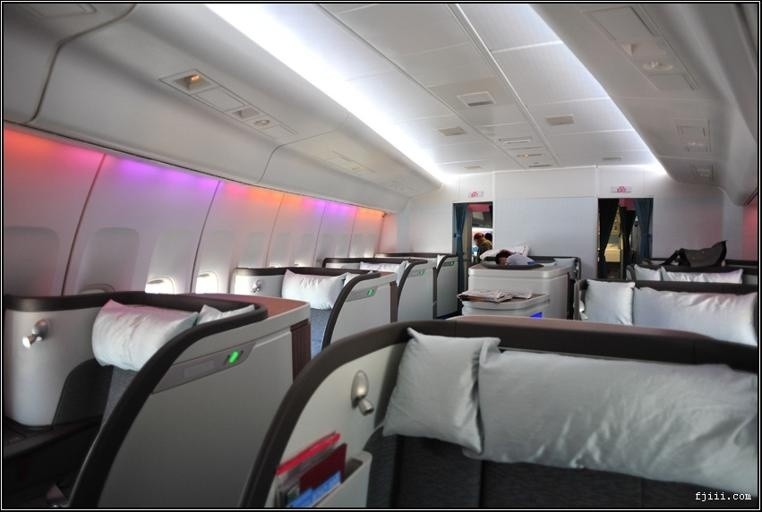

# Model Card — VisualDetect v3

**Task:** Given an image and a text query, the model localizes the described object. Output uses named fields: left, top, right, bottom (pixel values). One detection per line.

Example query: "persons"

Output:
left=497, top=250, right=535, bottom=265
left=473, top=233, right=492, bottom=263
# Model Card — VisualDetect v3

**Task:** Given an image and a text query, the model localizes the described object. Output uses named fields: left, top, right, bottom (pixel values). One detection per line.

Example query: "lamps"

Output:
left=21, top=319, right=46, bottom=349
left=350, top=369, right=374, bottom=417
left=250, top=280, right=261, bottom=294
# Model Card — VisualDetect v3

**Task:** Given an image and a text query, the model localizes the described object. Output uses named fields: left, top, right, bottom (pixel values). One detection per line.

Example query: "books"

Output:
left=276, top=433, right=348, bottom=508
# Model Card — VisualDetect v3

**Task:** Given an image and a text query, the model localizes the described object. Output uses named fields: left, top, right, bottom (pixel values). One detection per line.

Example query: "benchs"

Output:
left=569, top=275, right=759, bottom=348
left=374, top=250, right=461, bottom=319
left=228, top=267, right=398, bottom=359
left=244, top=314, right=758, bottom=510
left=642, top=257, right=757, bottom=266
left=624, top=264, right=757, bottom=288
left=323, top=258, right=425, bottom=321
left=1, top=293, right=313, bottom=510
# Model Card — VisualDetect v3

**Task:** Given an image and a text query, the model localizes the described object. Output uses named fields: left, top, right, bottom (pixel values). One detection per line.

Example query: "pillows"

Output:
left=356, top=259, right=409, bottom=288
left=634, top=286, right=757, bottom=349
left=381, top=325, right=501, bottom=454
left=634, top=264, right=661, bottom=284
left=344, top=270, right=373, bottom=289
left=463, top=337, right=757, bottom=497
left=662, top=268, right=744, bottom=284
left=281, top=269, right=346, bottom=312
left=436, top=254, right=447, bottom=269
left=90, top=299, right=198, bottom=372
left=197, top=303, right=256, bottom=324
left=583, top=278, right=634, bottom=329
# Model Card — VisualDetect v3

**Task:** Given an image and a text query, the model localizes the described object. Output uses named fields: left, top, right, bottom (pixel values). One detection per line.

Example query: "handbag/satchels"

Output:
left=645, top=241, right=727, bottom=267
